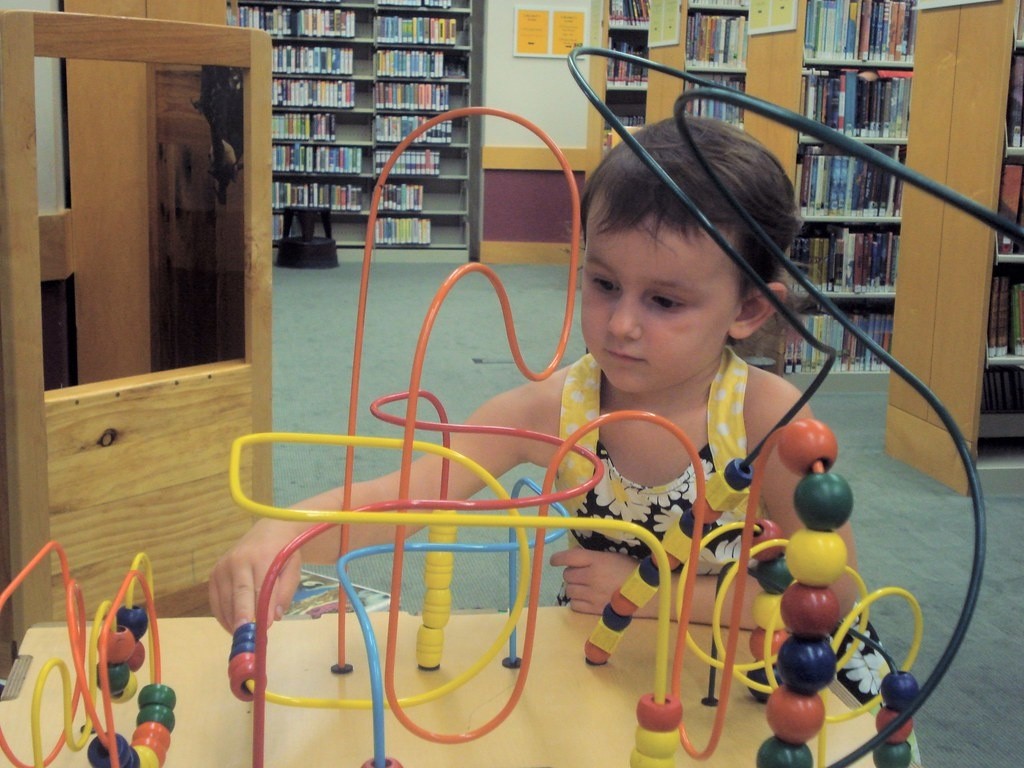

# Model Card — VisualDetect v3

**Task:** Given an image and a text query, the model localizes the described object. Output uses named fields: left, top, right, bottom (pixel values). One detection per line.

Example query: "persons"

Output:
left=196, top=63, right=245, bottom=178
left=209, top=115, right=862, bottom=638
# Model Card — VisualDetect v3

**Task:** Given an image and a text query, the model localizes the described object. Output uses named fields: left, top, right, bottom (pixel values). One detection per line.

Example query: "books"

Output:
left=229, top=0, right=457, bottom=245
left=604, top=0, right=650, bottom=159
left=784, top=0, right=917, bottom=375
left=980, top=0, right=1024, bottom=418
left=682, top=0, right=749, bottom=132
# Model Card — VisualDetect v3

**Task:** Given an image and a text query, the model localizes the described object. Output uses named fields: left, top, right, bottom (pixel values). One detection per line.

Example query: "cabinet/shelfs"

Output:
left=234, top=0, right=473, bottom=266
left=586, top=0, right=1023, bottom=498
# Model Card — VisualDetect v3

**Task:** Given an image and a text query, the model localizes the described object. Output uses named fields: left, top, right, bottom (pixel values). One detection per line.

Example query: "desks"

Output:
left=0, top=603, right=875, bottom=768
